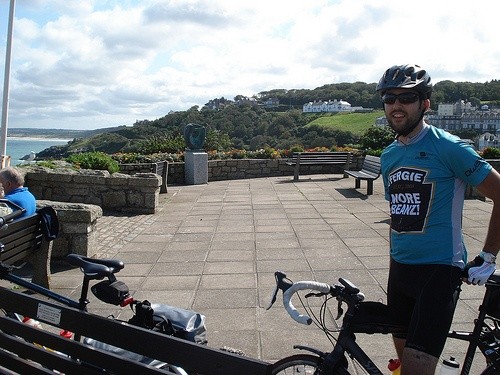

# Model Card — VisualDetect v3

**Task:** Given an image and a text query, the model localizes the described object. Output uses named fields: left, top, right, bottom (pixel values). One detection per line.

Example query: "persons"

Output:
left=375, top=64, right=500, bottom=375
left=0, top=167, right=36, bottom=220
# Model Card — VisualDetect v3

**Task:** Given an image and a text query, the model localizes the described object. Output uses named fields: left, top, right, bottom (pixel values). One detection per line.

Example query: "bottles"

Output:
left=22, top=316, right=54, bottom=353
left=387, top=358, right=401, bottom=375
left=53, top=328, right=73, bottom=375
left=438, top=356, right=460, bottom=375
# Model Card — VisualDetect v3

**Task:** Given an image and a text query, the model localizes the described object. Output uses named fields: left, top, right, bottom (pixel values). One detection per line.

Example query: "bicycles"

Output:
left=267, top=269, right=500, bottom=375
left=0, top=208, right=188, bottom=375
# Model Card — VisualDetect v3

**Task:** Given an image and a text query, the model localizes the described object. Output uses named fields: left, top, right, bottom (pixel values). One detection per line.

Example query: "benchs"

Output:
left=288, top=152, right=353, bottom=179
left=152, top=160, right=169, bottom=193
left=345, top=155, right=382, bottom=195
left=0, top=207, right=57, bottom=290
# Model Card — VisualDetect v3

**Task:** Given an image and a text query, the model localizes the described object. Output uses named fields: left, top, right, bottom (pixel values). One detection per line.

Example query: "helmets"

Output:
left=376, top=64, right=432, bottom=98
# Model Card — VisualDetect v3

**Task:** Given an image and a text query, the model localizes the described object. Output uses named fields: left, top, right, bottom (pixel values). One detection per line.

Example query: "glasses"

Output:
left=381, top=92, right=420, bottom=104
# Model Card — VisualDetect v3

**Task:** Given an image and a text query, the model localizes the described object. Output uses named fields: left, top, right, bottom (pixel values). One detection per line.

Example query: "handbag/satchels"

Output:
left=148, top=304, right=208, bottom=345
